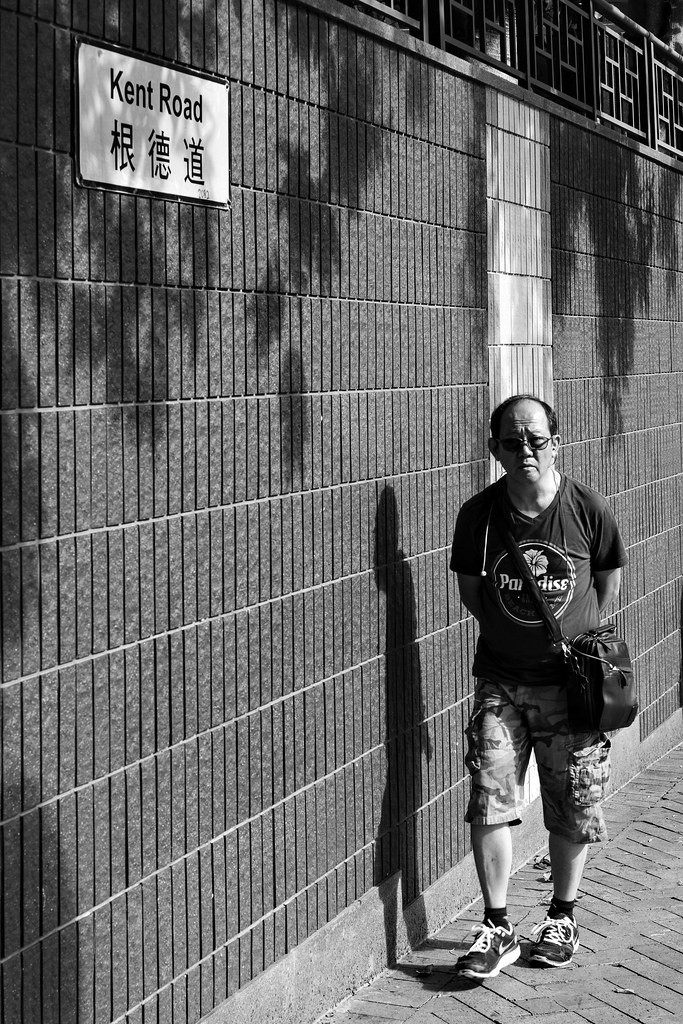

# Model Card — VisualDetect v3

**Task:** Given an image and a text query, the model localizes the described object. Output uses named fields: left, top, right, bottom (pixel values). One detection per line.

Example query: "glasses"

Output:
left=495, top=437, right=553, bottom=453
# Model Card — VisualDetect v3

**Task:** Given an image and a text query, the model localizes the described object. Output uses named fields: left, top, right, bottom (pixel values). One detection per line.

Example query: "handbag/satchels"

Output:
left=562, top=625, right=639, bottom=733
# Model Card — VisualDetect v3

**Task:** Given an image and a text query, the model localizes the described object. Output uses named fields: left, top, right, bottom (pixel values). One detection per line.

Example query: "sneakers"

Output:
left=528, top=914, right=579, bottom=967
left=456, top=917, right=521, bottom=979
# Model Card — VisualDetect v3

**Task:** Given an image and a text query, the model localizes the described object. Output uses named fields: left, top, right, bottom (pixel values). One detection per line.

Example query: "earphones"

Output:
left=553, top=447, right=555, bottom=450
left=480, top=571, right=486, bottom=576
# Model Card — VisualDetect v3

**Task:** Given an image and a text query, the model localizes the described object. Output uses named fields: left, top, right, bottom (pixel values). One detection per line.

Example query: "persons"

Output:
left=454, top=394, right=630, bottom=979
